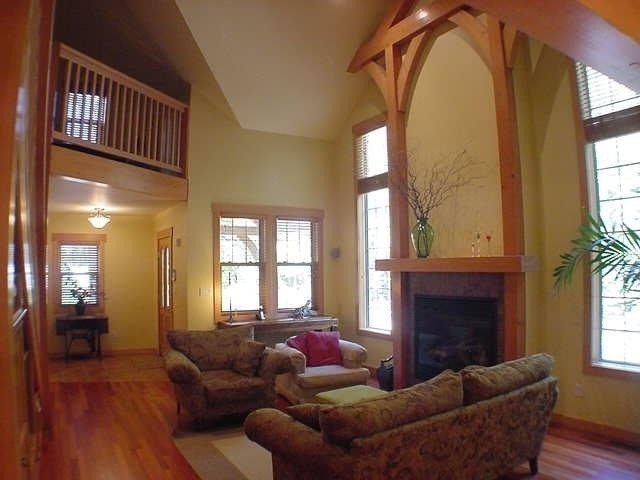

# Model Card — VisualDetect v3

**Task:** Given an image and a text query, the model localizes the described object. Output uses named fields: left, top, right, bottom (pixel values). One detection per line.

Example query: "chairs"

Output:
left=161, top=322, right=372, bottom=433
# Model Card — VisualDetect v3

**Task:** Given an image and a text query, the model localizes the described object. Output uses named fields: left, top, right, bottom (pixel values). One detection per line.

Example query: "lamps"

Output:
left=87, top=207, right=112, bottom=229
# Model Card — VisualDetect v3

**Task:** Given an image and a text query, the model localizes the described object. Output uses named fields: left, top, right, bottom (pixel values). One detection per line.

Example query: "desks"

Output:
left=55, top=314, right=110, bottom=361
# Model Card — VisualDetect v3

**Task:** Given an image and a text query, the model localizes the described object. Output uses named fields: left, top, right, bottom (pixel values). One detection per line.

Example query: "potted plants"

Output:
left=63, top=263, right=110, bottom=316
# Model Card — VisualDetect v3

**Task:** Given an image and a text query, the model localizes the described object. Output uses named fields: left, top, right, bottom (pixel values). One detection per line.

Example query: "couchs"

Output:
left=243, top=351, right=561, bottom=479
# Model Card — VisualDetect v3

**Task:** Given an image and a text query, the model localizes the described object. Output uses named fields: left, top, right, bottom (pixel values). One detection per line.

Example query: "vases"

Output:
left=410, top=216, right=435, bottom=259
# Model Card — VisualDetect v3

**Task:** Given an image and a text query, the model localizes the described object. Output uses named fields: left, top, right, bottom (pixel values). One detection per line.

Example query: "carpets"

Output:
left=170, top=426, right=274, bottom=480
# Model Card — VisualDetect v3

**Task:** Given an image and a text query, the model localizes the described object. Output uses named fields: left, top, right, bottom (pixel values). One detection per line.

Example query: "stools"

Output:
left=310, top=383, right=391, bottom=405
